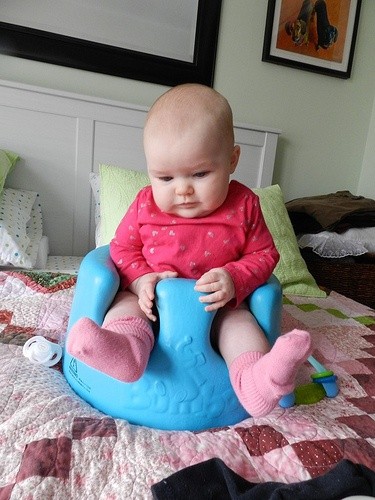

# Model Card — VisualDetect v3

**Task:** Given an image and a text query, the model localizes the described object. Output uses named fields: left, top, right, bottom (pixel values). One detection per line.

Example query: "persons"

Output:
left=66, top=83, right=312, bottom=418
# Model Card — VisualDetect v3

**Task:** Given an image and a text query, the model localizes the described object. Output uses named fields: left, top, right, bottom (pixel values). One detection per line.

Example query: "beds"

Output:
left=0, top=80, right=375, bottom=500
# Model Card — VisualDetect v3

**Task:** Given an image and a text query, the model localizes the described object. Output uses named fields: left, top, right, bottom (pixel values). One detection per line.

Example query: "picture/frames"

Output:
left=0, top=0, right=223, bottom=89
left=261, top=0, right=362, bottom=80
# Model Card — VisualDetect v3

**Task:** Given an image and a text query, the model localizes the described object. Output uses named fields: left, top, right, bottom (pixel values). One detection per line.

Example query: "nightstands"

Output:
left=306, top=257, right=375, bottom=311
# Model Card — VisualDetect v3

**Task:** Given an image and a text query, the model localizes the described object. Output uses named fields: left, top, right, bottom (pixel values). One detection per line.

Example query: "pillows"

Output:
left=0, top=149, right=375, bottom=298
